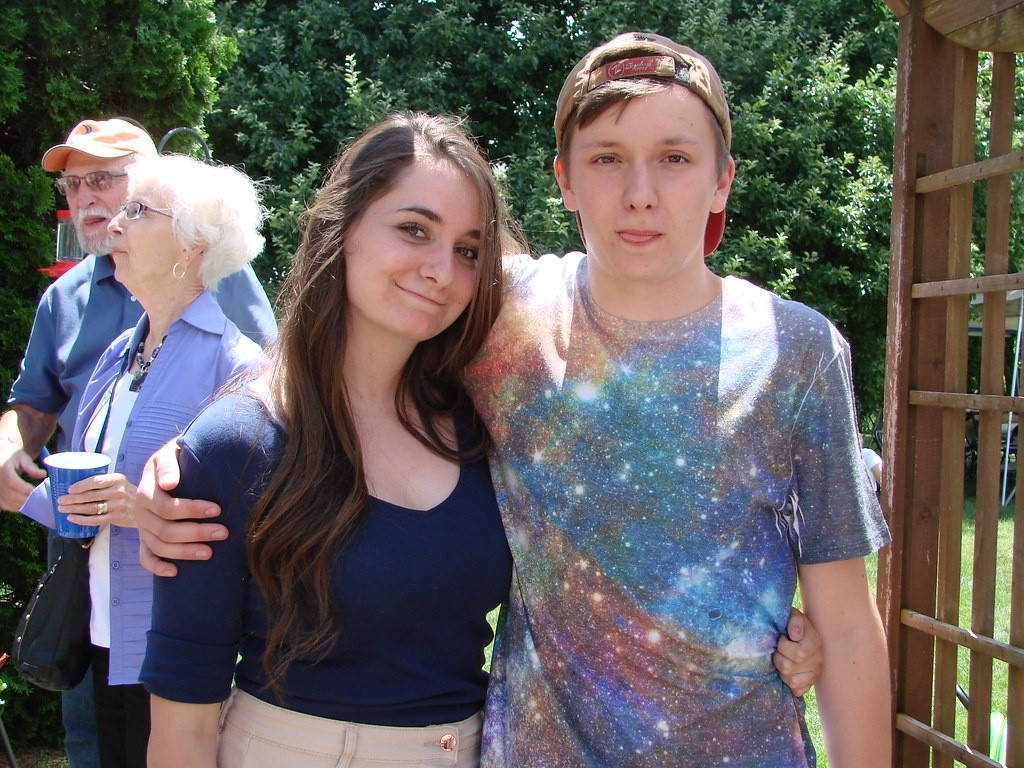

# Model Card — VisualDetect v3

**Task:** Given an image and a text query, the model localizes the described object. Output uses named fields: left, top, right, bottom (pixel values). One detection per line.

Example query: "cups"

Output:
left=43, top=452, right=108, bottom=539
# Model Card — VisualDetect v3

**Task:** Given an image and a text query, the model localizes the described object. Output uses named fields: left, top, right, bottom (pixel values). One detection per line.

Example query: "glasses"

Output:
left=120, top=201, right=174, bottom=220
left=54, top=171, right=128, bottom=195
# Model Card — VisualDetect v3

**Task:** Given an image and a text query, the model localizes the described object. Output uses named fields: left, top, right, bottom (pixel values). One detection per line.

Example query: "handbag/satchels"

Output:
left=11, top=541, right=89, bottom=692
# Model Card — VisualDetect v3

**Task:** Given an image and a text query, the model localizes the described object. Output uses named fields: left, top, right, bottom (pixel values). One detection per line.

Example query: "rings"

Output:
left=97, top=502, right=104, bottom=515
left=103, top=501, right=108, bottom=514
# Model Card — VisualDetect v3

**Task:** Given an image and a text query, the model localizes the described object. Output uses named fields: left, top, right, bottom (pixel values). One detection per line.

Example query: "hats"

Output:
left=554, top=32, right=729, bottom=258
left=42, top=119, right=158, bottom=172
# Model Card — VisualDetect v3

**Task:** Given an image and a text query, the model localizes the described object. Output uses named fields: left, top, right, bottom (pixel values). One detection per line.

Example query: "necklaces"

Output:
left=129, top=322, right=168, bottom=392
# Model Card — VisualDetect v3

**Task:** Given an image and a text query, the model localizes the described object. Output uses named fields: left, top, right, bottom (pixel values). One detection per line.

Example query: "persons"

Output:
left=0, top=32, right=893, bottom=768
